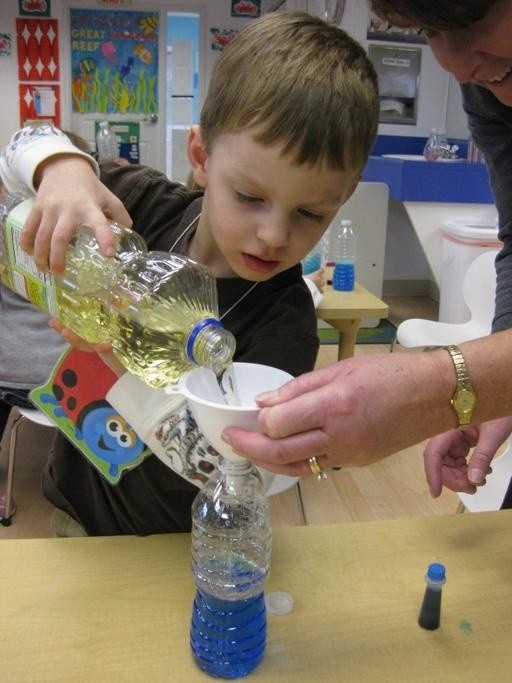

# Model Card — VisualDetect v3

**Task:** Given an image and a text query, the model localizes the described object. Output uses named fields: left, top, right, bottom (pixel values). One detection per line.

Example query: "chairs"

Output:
left=262, top=473, right=309, bottom=526
left=388, top=250, right=500, bottom=353
left=455, top=432, right=512, bottom=514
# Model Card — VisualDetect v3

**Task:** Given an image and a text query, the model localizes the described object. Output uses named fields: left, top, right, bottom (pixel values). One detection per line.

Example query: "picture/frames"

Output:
left=368, top=44, right=422, bottom=125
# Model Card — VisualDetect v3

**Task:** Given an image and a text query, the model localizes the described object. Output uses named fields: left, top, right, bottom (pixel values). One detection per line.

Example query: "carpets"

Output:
left=317, top=318, right=398, bottom=345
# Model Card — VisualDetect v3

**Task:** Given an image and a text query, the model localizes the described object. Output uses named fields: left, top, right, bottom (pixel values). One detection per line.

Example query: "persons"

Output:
left=302, top=267, right=325, bottom=310
left=0, top=9, right=379, bottom=538
left=219, top=0, right=509, bottom=514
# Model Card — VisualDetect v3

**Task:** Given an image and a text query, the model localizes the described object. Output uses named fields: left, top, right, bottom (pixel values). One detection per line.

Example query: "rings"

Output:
left=307, top=456, right=327, bottom=481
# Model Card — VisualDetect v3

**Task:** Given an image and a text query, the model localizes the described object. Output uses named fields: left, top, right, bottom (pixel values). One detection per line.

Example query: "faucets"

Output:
left=436, top=145, right=459, bottom=159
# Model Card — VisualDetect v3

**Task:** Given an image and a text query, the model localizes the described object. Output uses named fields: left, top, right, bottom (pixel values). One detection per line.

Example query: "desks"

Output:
left=0, top=509, right=512, bottom=683
left=359, top=156, right=500, bottom=290
left=315, top=263, right=389, bottom=362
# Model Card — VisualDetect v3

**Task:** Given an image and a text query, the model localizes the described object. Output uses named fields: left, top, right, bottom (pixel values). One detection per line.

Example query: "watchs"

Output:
left=432, top=345, right=476, bottom=429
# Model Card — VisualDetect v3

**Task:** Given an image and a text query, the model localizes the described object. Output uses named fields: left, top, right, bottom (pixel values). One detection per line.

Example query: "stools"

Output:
left=0, top=404, right=58, bottom=527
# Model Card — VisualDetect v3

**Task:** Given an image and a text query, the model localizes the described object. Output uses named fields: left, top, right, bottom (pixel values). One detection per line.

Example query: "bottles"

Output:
left=191, top=456, right=272, bottom=678
left=418, top=562, right=446, bottom=630
left=96, top=120, right=119, bottom=161
left=422, top=126, right=455, bottom=162
left=0, top=183, right=236, bottom=390
left=301, top=219, right=357, bottom=291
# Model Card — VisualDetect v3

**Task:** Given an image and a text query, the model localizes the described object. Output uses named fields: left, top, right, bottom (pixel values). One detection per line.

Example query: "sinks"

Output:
left=382, top=154, right=466, bottom=162
